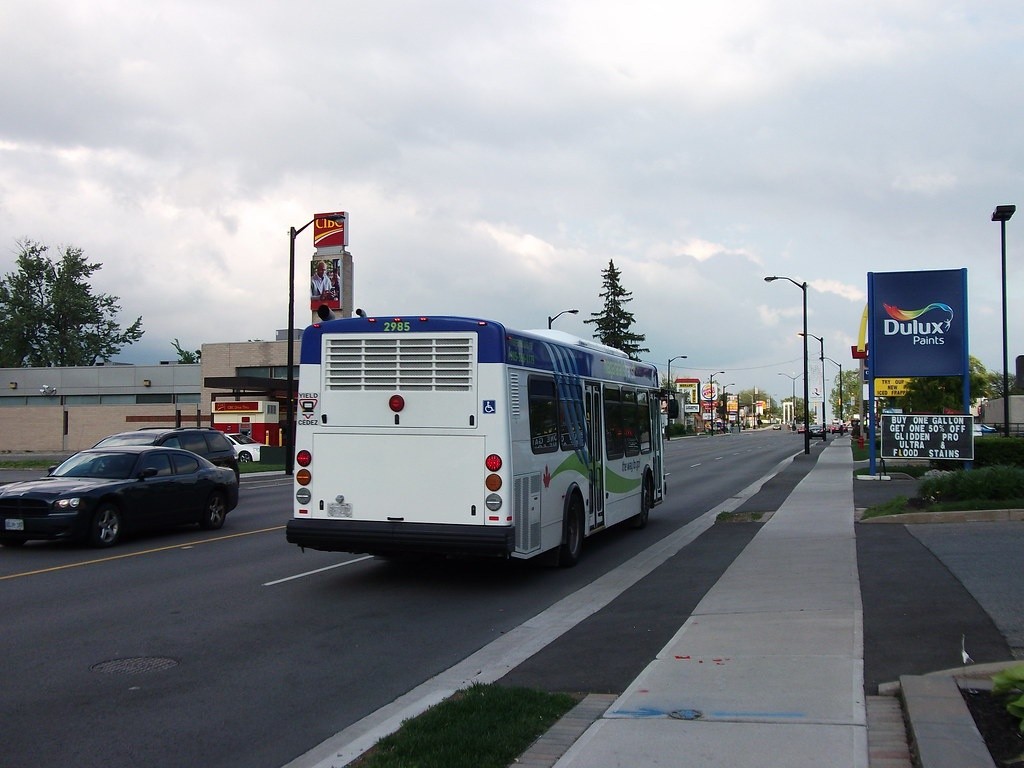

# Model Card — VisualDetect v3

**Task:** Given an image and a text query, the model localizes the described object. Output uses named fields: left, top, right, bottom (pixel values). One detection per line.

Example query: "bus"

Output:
left=286, top=316, right=679, bottom=569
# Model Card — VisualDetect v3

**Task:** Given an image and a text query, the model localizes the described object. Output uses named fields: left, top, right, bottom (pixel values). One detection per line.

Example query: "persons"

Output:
left=835, top=423, right=840, bottom=430
left=840, top=425, right=843, bottom=436
left=792, top=424, right=796, bottom=431
left=741, top=419, right=745, bottom=430
left=809, top=428, right=813, bottom=439
left=311, top=261, right=332, bottom=296
left=705, top=420, right=734, bottom=434
left=757, top=419, right=763, bottom=428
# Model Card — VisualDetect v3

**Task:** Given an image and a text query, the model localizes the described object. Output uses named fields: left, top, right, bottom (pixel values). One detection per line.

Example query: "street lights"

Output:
left=723, top=383, right=735, bottom=434
left=738, top=403, right=755, bottom=432
left=778, top=372, right=804, bottom=431
left=770, top=394, right=777, bottom=424
left=710, top=372, right=725, bottom=436
left=754, top=393, right=766, bottom=429
left=797, top=332, right=826, bottom=440
left=764, top=276, right=810, bottom=455
left=548, top=310, right=579, bottom=330
left=819, top=356, right=844, bottom=437
left=666, top=356, right=686, bottom=439
left=991, top=204, right=1017, bottom=438
left=285, top=215, right=345, bottom=475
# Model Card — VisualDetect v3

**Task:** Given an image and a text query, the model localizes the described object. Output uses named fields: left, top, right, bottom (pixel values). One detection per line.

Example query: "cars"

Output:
left=825, top=423, right=848, bottom=434
left=713, top=419, right=726, bottom=428
left=0, top=445, right=239, bottom=548
left=810, top=425, right=824, bottom=440
left=788, top=422, right=792, bottom=427
left=224, top=433, right=271, bottom=463
left=798, top=426, right=805, bottom=433
left=801, top=421, right=805, bottom=426
left=773, top=425, right=782, bottom=430
left=91, top=427, right=240, bottom=487
left=982, top=425, right=996, bottom=433
